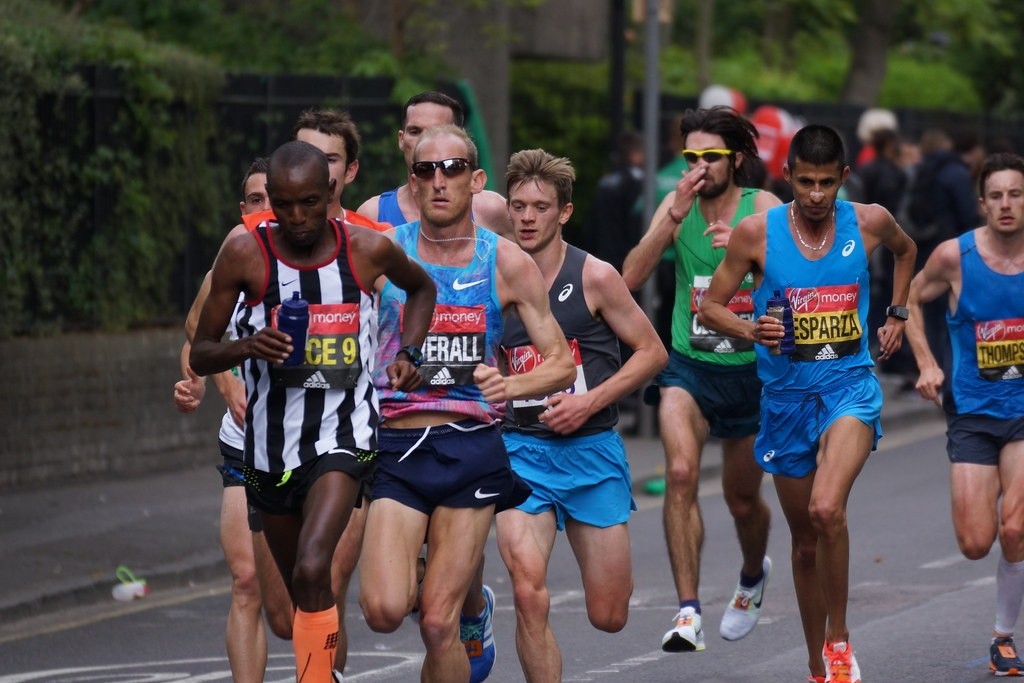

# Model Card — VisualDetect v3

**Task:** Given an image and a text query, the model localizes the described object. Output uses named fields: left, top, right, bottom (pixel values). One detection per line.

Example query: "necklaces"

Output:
left=790, top=199, right=835, bottom=251
left=420, top=220, right=491, bottom=261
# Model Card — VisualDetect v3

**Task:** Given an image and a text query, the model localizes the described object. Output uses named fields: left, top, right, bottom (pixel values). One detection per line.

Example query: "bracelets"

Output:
left=668, top=207, right=683, bottom=224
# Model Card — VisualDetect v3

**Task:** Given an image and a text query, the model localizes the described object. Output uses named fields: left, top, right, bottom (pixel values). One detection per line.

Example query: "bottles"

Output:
left=764, top=290, right=797, bottom=357
left=110, top=576, right=149, bottom=600
left=277, top=288, right=307, bottom=368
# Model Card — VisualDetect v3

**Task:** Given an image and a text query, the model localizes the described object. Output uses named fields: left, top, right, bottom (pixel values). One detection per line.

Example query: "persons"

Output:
left=171, top=91, right=668, bottom=683
left=904, top=149, right=1023, bottom=676
left=620, top=110, right=787, bottom=653
left=653, top=83, right=1024, bottom=420
left=696, top=124, right=919, bottom=683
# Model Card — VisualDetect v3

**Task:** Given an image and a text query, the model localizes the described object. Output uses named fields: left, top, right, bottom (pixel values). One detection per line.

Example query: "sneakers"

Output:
left=719, top=556, right=773, bottom=642
left=661, top=607, right=706, bottom=653
left=460, top=584, right=495, bottom=681
left=807, top=640, right=861, bottom=683
left=989, top=637, right=1024, bottom=676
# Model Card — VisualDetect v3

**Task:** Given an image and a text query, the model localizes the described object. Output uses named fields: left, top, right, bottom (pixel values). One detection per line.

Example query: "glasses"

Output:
left=681, top=148, right=733, bottom=164
left=411, top=158, right=471, bottom=179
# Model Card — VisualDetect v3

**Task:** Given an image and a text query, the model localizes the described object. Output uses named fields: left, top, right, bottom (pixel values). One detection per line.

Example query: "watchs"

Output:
left=396, top=345, right=424, bottom=368
left=886, top=305, right=909, bottom=321
